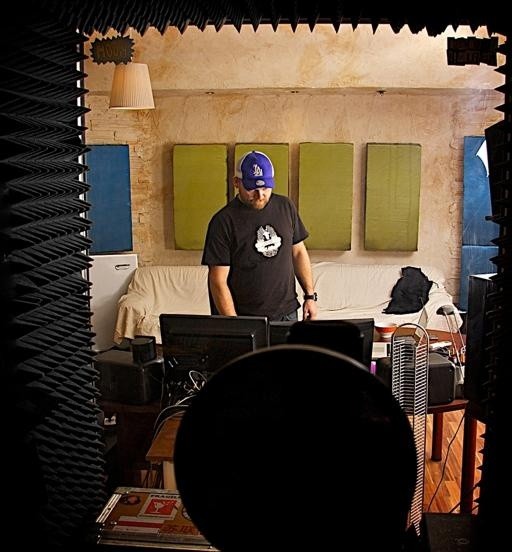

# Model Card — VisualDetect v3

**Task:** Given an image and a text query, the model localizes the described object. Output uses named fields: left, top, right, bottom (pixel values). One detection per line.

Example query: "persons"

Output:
left=200, top=150, right=317, bottom=323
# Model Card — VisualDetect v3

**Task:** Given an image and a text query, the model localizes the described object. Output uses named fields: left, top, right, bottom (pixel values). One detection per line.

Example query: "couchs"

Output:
left=119, top=262, right=463, bottom=355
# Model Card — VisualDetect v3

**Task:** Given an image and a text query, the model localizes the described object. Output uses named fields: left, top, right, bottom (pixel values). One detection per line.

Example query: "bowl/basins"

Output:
left=374, top=322, right=398, bottom=339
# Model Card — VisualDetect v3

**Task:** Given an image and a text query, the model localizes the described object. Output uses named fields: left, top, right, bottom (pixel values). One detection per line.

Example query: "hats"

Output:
left=236, top=149, right=276, bottom=189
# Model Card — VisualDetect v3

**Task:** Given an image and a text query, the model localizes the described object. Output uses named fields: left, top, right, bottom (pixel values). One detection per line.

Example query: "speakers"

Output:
left=377, top=353, right=454, bottom=406
left=93, top=350, right=164, bottom=405
left=466, top=272, right=499, bottom=423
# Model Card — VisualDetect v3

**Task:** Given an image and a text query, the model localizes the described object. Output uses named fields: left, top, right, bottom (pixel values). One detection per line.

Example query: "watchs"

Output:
left=303, top=292, right=318, bottom=302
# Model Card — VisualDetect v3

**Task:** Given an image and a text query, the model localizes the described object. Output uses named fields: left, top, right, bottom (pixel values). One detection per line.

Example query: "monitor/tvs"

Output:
left=159, top=314, right=269, bottom=382
left=269, top=318, right=374, bottom=369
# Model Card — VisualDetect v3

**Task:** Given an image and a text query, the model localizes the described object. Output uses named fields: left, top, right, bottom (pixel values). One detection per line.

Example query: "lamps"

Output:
left=107, top=63, right=156, bottom=114
